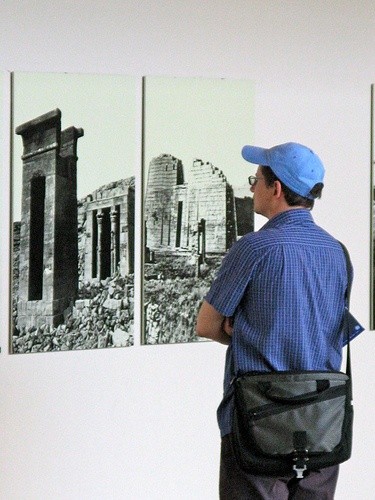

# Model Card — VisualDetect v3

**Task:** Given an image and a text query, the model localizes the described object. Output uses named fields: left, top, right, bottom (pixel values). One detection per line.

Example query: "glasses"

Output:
left=247, top=174, right=266, bottom=185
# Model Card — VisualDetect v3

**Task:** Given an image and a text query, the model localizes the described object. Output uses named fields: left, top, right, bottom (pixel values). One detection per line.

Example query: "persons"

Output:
left=197, top=141, right=354, bottom=500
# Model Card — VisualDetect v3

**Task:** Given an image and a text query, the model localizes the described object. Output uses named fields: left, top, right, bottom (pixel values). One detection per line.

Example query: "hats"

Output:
left=242, top=142, right=326, bottom=202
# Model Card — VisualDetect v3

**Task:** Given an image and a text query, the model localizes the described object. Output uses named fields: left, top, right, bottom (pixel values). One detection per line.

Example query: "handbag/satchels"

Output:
left=229, top=368, right=355, bottom=478
